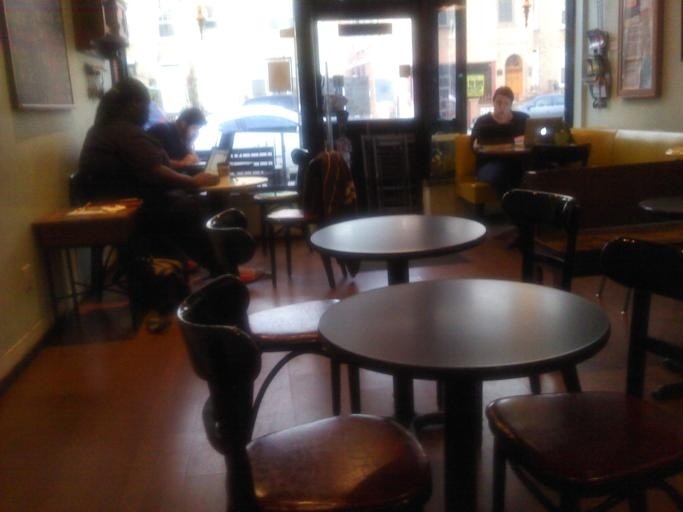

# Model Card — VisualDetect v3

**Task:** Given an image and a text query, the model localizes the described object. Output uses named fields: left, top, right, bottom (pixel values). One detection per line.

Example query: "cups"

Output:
left=216, top=161, right=230, bottom=185
left=554, top=131, right=568, bottom=147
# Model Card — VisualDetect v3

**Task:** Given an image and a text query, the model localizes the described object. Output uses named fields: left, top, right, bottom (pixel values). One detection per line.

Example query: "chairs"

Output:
left=510, top=141, right=593, bottom=252
left=484, top=232, right=682, bottom=512
left=208, top=209, right=343, bottom=439
left=505, top=190, right=587, bottom=397
left=77, top=150, right=323, bottom=276
left=178, top=274, right=432, bottom=512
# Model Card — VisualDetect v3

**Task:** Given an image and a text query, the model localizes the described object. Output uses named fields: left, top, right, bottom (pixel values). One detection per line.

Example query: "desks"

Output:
left=315, top=278, right=611, bottom=511
left=310, top=214, right=489, bottom=431
left=37, top=201, right=152, bottom=334
left=475, top=138, right=583, bottom=198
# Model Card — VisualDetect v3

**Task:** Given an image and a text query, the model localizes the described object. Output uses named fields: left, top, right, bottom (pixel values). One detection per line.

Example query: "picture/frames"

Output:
left=615, top=0, right=664, bottom=98
left=0, top=0, right=76, bottom=113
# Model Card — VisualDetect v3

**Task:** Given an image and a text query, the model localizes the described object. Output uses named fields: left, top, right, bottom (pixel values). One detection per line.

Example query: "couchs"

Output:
left=455, top=129, right=681, bottom=214
left=523, top=161, right=679, bottom=278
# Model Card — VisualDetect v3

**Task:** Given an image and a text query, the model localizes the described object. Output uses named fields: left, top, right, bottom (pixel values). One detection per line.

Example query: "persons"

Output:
left=146, top=107, right=209, bottom=200
left=469, top=86, right=530, bottom=183
left=145, top=77, right=164, bottom=112
left=79, top=75, right=267, bottom=284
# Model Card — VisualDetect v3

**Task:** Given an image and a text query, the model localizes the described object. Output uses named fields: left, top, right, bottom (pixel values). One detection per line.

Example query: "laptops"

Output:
left=525, top=117, right=562, bottom=146
left=202, top=147, right=230, bottom=177
